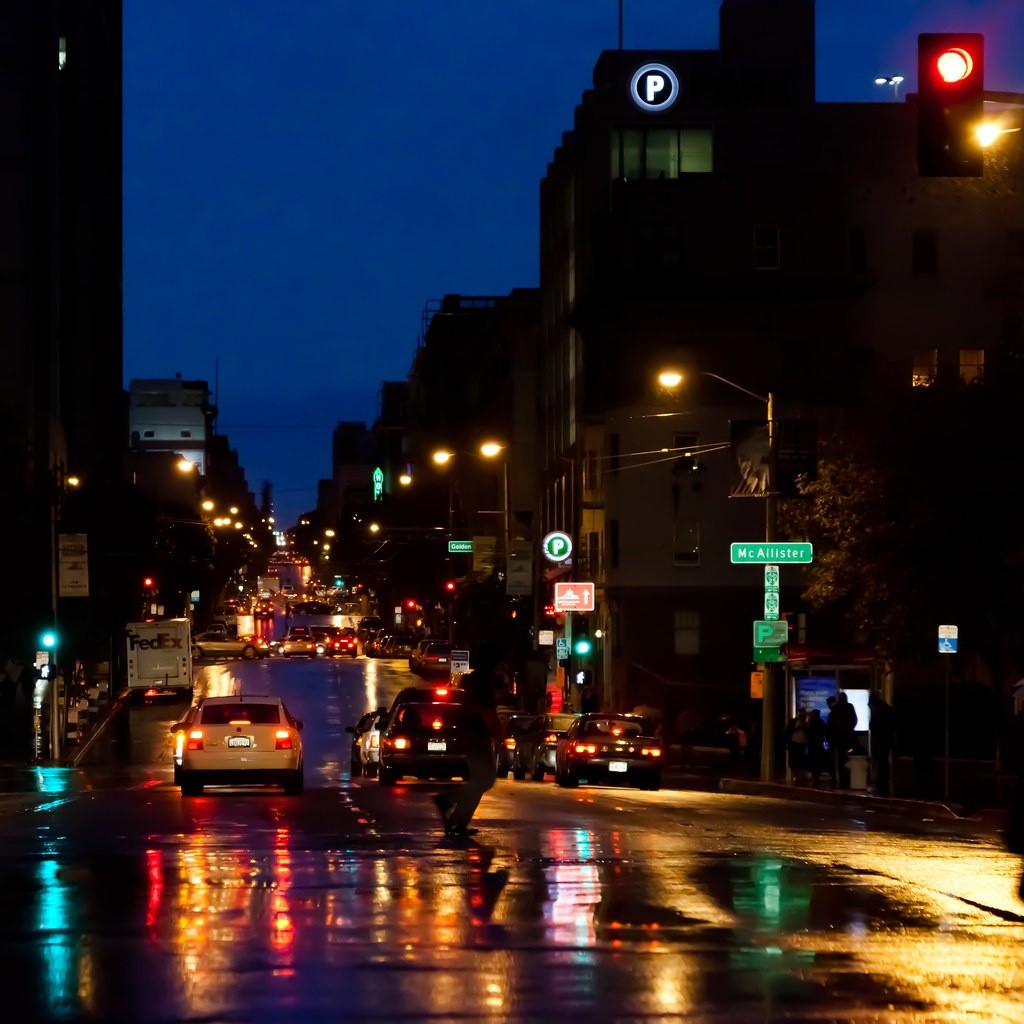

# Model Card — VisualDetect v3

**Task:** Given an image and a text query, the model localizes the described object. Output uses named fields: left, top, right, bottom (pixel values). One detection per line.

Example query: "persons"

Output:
left=436, top=663, right=513, bottom=835
left=787, top=692, right=857, bottom=790
left=581, top=687, right=599, bottom=714
left=868, top=690, right=896, bottom=798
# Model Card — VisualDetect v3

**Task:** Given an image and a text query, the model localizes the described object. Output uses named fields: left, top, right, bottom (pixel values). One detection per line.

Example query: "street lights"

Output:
left=398, top=471, right=456, bottom=644
left=428, top=445, right=523, bottom=714
left=478, top=435, right=583, bottom=715
left=653, top=363, right=775, bottom=783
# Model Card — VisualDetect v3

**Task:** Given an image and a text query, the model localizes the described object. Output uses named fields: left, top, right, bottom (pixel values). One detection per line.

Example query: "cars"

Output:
left=409, top=638, right=455, bottom=673
left=345, top=711, right=391, bottom=778
left=190, top=552, right=422, bottom=663
left=419, top=641, right=470, bottom=679
left=554, top=712, right=667, bottom=790
left=375, top=686, right=580, bottom=785
left=169, top=692, right=305, bottom=797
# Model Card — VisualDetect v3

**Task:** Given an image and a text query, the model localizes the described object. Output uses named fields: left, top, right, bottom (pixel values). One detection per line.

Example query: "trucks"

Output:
left=124, top=618, right=196, bottom=700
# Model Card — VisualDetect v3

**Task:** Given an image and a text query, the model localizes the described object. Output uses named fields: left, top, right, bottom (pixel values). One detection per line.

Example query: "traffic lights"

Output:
left=403, top=597, right=418, bottom=615
left=545, top=604, right=562, bottom=620
left=913, top=28, right=987, bottom=180
left=143, top=574, right=155, bottom=593
left=445, top=576, right=457, bottom=602
left=39, top=626, right=57, bottom=666
left=574, top=668, right=593, bottom=687
left=575, top=631, right=593, bottom=662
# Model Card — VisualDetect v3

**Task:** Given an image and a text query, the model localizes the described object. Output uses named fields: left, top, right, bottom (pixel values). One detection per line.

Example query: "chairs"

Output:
left=585, top=721, right=601, bottom=738
left=623, top=727, right=641, bottom=739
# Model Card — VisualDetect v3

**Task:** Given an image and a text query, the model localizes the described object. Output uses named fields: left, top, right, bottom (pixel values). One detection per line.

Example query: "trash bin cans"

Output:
left=850, top=756, right=869, bottom=789
